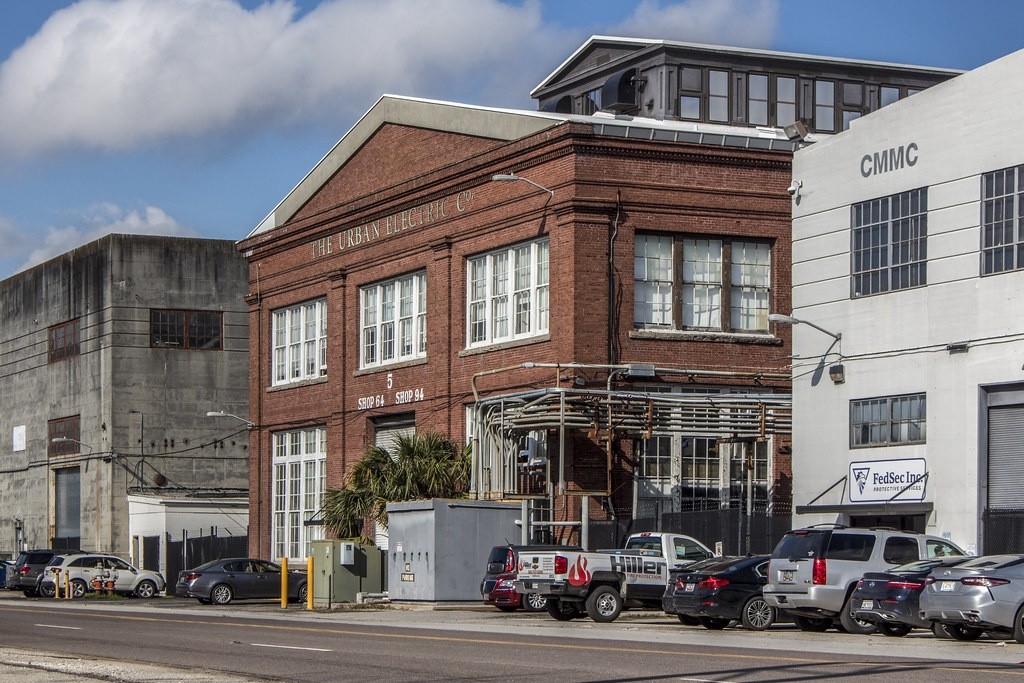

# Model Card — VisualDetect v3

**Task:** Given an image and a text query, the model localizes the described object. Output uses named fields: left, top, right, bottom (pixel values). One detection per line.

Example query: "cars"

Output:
left=850, top=555, right=984, bottom=637
left=919, top=554, right=1024, bottom=644
left=175, top=558, right=307, bottom=606
left=661, top=554, right=774, bottom=630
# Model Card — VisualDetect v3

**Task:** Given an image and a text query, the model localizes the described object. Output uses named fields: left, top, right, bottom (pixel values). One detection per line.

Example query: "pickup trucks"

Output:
left=512, top=532, right=720, bottom=623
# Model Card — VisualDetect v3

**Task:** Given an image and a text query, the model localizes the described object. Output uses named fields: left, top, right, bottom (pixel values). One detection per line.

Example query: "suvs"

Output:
left=40, top=554, right=167, bottom=599
left=12, top=549, right=95, bottom=598
left=762, top=522, right=969, bottom=634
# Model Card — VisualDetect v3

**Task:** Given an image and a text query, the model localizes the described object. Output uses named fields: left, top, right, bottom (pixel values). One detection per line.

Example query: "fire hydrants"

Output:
left=103, top=566, right=119, bottom=597
left=90, top=563, right=104, bottom=598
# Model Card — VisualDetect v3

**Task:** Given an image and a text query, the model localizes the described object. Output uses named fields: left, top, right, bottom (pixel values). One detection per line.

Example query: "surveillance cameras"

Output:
left=787, top=186, right=796, bottom=194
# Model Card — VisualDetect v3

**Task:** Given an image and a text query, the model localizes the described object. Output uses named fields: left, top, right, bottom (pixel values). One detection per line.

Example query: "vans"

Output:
left=480, top=538, right=586, bottom=612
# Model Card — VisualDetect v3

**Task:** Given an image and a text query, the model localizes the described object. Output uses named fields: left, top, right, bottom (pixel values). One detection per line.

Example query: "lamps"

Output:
left=492, top=174, right=554, bottom=196
left=767, top=314, right=841, bottom=340
left=783, top=121, right=808, bottom=151
left=52, top=438, right=92, bottom=449
left=829, top=364, right=845, bottom=382
left=206, top=412, right=255, bottom=429
left=631, top=76, right=648, bottom=81
